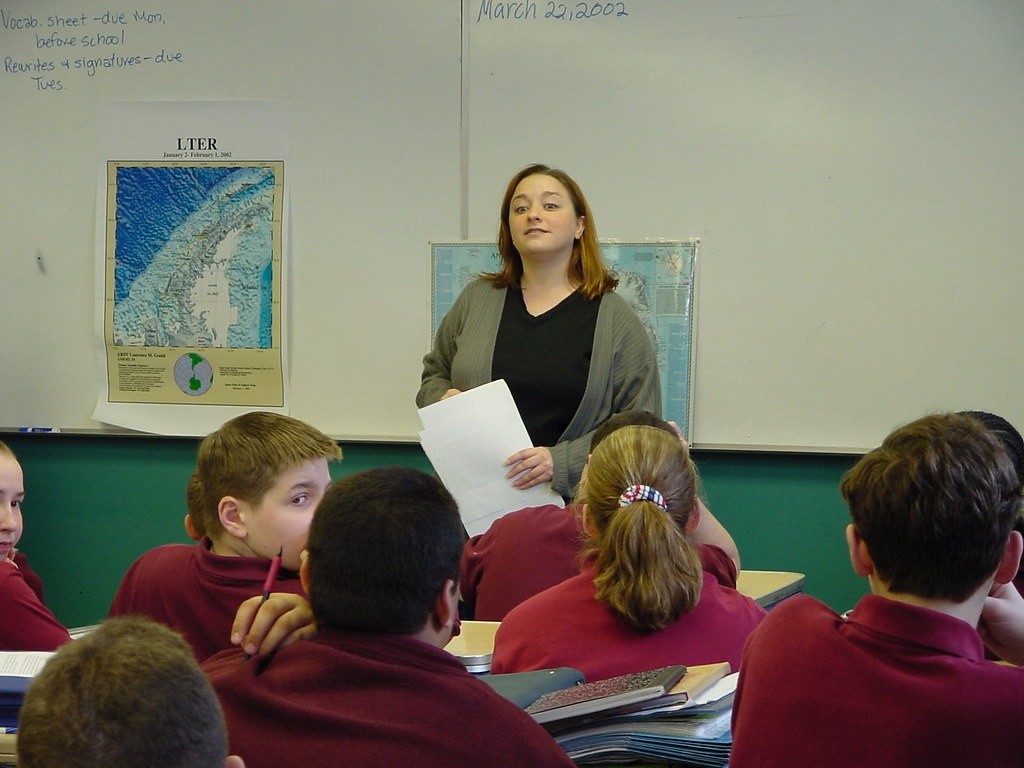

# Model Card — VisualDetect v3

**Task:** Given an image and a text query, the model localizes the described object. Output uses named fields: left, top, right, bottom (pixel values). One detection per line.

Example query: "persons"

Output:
left=200, top=464, right=576, bottom=768
left=0, top=441, right=74, bottom=652
left=15, top=617, right=247, bottom=768
left=106, top=410, right=344, bottom=661
left=183, top=469, right=207, bottom=541
left=455, top=410, right=741, bottom=623
left=725, top=413, right=1024, bottom=768
left=415, top=162, right=664, bottom=508
left=490, top=423, right=770, bottom=683
left=958, top=409, right=1024, bottom=661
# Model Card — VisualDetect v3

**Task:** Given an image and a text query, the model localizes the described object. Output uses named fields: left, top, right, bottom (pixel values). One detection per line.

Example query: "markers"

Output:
left=18, top=426, right=60, bottom=433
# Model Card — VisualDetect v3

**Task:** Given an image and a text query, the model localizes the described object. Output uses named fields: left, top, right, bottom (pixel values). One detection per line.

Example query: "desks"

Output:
left=737, top=571, right=808, bottom=608
left=444, top=621, right=503, bottom=673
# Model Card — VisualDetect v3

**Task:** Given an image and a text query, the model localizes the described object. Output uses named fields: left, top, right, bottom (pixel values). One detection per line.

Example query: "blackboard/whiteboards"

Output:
left=0, top=0, right=1024, bottom=457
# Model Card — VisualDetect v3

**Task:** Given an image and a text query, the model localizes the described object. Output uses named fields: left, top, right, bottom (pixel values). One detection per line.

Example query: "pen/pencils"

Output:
left=243, top=545, right=284, bottom=662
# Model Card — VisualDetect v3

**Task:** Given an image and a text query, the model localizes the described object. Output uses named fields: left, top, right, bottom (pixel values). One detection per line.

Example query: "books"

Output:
left=467, top=661, right=733, bottom=768
